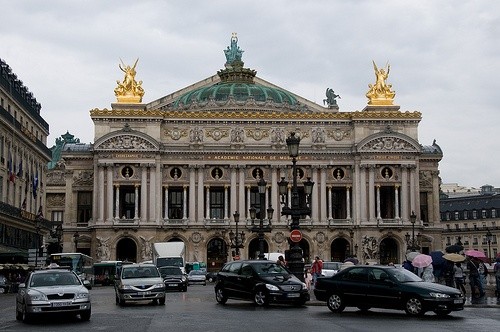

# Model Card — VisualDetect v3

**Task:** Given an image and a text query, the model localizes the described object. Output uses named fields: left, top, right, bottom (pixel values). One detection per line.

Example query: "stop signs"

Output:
left=290, top=230, right=302, bottom=243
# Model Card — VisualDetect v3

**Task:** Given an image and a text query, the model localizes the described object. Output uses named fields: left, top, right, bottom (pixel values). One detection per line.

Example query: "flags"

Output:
left=10, top=173, right=38, bottom=199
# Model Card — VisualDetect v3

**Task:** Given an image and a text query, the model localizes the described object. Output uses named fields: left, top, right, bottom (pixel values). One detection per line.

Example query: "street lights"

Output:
left=229, top=210, right=245, bottom=261
left=485, top=229, right=492, bottom=266
left=249, top=176, right=274, bottom=260
left=405, top=210, right=422, bottom=273
left=276, top=131, right=316, bottom=282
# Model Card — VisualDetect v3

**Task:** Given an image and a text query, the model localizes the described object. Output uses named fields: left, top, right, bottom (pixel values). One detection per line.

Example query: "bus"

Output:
left=45, top=253, right=94, bottom=290
left=93, top=261, right=137, bottom=287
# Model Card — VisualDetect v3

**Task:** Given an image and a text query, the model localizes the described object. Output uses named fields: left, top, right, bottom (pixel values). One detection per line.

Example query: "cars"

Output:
left=313, top=265, right=466, bottom=319
left=214, top=259, right=310, bottom=309
left=16, top=269, right=92, bottom=326
left=206, top=271, right=219, bottom=283
left=113, top=263, right=167, bottom=307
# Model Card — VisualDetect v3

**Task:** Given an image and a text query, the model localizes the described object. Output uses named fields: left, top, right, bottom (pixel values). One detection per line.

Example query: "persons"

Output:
left=224, top=36, right=244, bottom=61
left=402, top=259, right=500, bottom=302
left=114, top=64, right=145, bottom=96
left=365, top=61, right=396, bottom=99
left=278, top=256, right=322, bottom=290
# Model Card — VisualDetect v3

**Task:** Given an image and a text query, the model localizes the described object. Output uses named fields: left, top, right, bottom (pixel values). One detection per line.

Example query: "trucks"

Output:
left=151, top=241, right=190, bottom=292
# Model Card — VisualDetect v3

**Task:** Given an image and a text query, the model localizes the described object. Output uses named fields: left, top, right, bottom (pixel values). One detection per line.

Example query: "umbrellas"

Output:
left=406, top=245, right=500, bottom=269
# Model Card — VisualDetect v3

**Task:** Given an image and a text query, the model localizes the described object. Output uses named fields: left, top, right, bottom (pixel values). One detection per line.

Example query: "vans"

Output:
left=185, top=262, right=207, bottom=286
left=320, top=262, right=342, bottom=279
